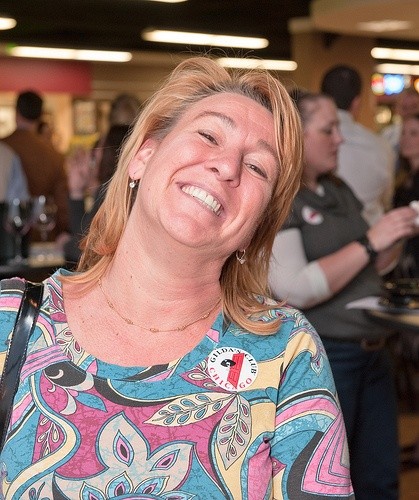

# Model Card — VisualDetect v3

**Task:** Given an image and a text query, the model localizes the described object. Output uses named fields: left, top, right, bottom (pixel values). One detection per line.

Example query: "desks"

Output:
left=0, top=241, right=65, bottom=276
left=363, top=293, right=419, bottom=469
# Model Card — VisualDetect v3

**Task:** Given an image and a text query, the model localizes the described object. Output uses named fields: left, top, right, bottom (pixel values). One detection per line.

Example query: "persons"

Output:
left=320, top=61, right=419, bottom=278
left=1, top=54, right=356, bottom=500
left=263, top=91, right=419, bottom=498
left=1, top=64, right=142, bottom=270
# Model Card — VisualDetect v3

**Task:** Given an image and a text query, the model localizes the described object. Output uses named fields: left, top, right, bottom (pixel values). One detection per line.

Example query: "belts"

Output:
left=321, top=334, right=389, bottom=352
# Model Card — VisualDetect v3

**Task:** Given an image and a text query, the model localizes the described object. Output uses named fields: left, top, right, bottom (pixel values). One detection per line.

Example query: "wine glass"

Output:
left=31, top=197, right=57, bottom=260
left=3, top=201, right=29, bottom=266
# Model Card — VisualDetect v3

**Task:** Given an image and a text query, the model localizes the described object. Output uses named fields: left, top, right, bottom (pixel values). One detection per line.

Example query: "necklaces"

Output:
left=96, top=278, right=223, bottom=333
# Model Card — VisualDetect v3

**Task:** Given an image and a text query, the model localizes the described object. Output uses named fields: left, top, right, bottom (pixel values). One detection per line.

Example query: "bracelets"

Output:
left=354, top=233, right=379, bottom=262
